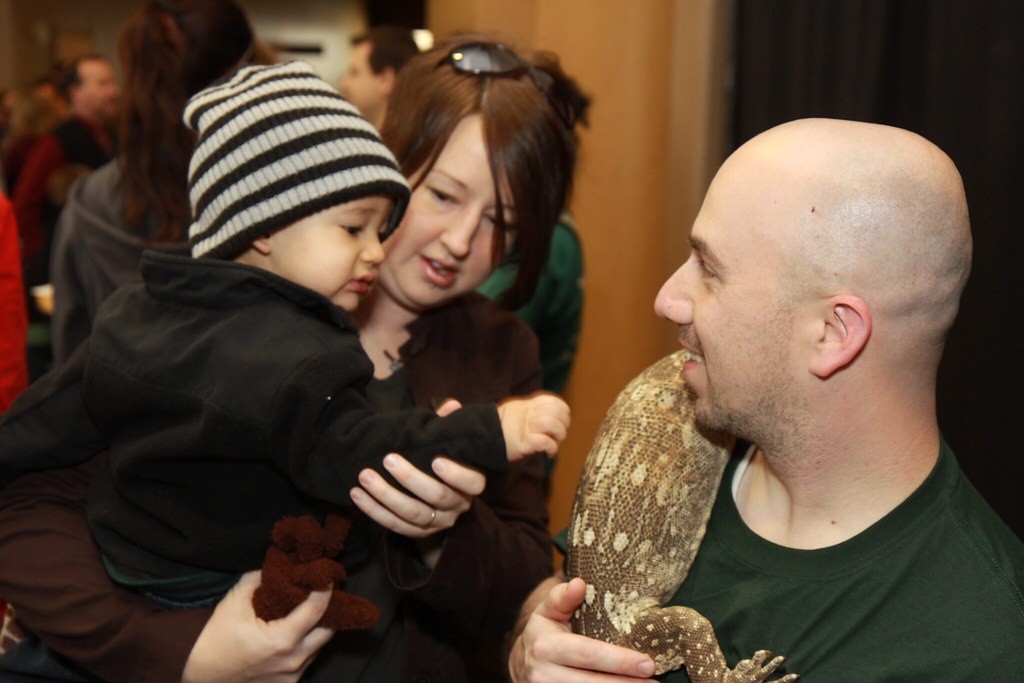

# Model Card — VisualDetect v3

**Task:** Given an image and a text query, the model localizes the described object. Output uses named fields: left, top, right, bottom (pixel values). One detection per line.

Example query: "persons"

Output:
left=502, top=118, right=1024, bottom=683
left=0, top=0, right=592, bottom=683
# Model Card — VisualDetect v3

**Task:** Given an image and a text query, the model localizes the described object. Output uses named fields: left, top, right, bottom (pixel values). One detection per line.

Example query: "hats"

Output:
left=184, top=60, right=412, bottom=263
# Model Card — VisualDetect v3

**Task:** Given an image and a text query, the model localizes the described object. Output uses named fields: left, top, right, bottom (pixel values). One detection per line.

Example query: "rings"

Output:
left=423, top=509, right=435, bottom=530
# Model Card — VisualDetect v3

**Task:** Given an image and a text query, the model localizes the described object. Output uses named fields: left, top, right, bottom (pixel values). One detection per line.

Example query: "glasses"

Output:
left=435, top=41, right=576, bottom=135
